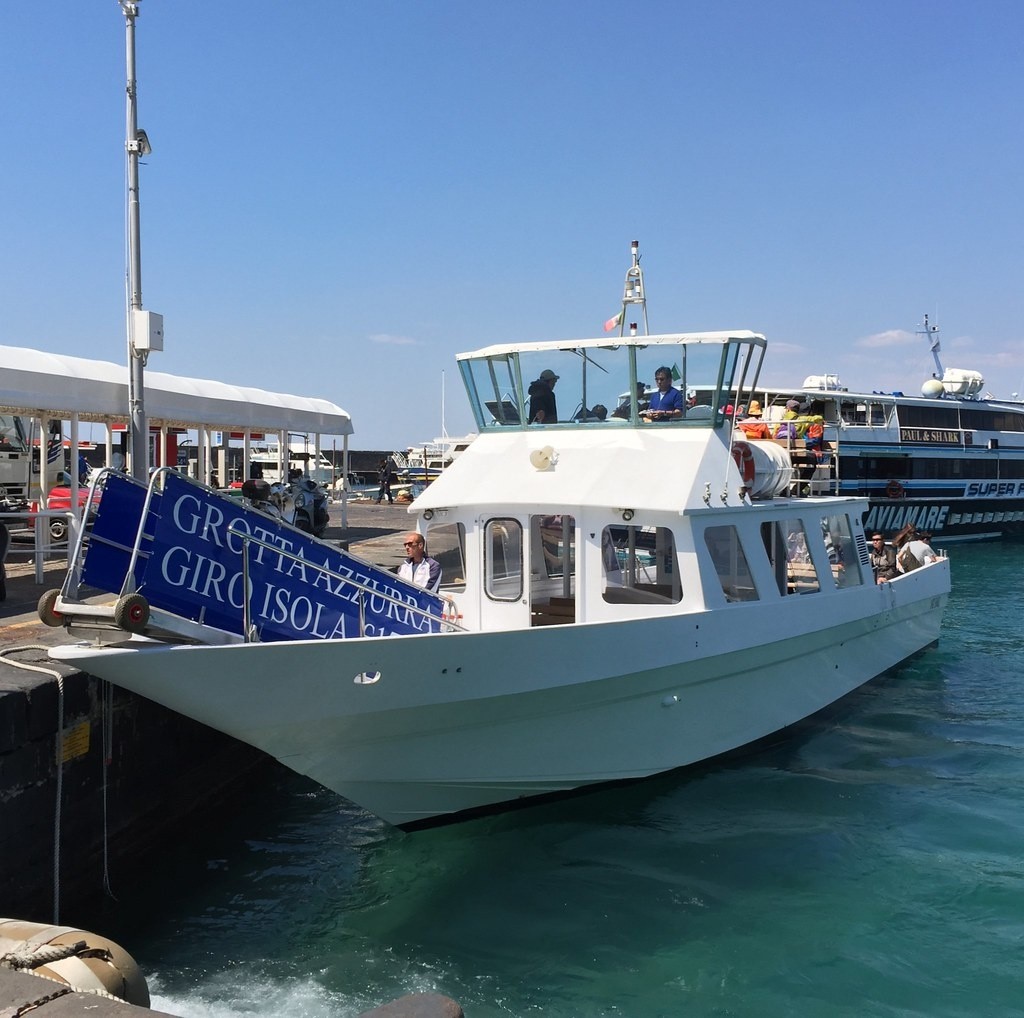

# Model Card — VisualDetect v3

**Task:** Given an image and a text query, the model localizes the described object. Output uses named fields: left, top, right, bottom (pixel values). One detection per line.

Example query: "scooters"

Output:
left=242, top=469, right=329, bottom=538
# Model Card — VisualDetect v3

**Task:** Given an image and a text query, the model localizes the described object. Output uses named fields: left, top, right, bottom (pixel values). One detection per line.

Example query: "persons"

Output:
left=374, top=458, right=393, bottom=505
left=645, top=367, right=684, bottom=422
left=396, top=534, right=442, bottom=594
left=897, top=531, right=937, bottom=576
left=802, top=399, right=832, bottom=465
left=772, top=399, right=823, bottom=498
left=618, top=382, right=645, bottom=419
left=527, top=369, right=559, bottom=424
left=738, top=400, right=770, bottom=439
left=870, top=532, right=900, bottom=584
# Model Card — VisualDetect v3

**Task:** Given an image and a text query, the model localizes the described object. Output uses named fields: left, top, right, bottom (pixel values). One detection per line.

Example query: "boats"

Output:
left=49, top=240, right=951, bottom=833
left=391, top=433, right=478, bottom=485
left=250, top=443, right=340, bottom=485
left=609, top=314, right=1023, bottom=542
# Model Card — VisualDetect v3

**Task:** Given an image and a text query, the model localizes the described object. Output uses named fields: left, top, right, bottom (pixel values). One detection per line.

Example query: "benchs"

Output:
left=786, top=563, right=839, bottom=593
left=746, top=438, right=816, bottom=497
left=813, top=440, right=839, bottom=494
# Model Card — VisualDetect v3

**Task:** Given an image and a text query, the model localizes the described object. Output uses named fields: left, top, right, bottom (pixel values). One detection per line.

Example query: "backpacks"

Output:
left=776, top=415, right=799, bottom=439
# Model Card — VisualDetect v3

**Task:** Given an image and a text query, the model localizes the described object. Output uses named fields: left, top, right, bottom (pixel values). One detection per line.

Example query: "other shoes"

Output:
left=388, top=501, right=393, bottom=504
left=791, top=485, right=808, bottom=498
left=779, top=487, right=790, bottom=497
left=372, top=500, right=380, bottom=505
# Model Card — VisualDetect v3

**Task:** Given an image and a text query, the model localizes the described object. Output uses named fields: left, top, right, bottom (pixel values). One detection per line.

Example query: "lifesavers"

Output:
left=729, top=441, right=756, bottom=490
left=886, top=480, right=904, bottom=499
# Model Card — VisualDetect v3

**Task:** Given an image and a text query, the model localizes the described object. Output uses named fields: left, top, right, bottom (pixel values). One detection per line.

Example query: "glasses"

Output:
left=404, top=541, right=420, bottom=547
left=654, top=377, right=667, bottom=382
left=872, top=538, right=881, bottom=542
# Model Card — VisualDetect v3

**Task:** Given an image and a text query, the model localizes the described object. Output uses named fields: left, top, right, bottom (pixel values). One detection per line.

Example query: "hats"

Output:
left=747, top=400, right=764, bottom=416
left=340, top=473, right=343, bottom=476
left=720, top=404, right=734, bottom=415
left=784, top=400, right=800, bottom=412
left=734, top=405, right=749, bottom=417
left=637, top=382, right=645, bottom=389
left=540, top=369, right=560, bottom=381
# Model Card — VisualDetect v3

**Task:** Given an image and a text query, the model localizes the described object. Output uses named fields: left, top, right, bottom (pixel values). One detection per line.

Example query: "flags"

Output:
left=670, top=363, right=682, bottom=381
left=930, top=338, right=941, bottom=353
left=604, top=312, right=622, bottom=331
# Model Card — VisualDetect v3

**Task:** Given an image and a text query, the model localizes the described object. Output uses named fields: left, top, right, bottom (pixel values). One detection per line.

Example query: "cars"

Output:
left=25, top=489, right=102, bottom=542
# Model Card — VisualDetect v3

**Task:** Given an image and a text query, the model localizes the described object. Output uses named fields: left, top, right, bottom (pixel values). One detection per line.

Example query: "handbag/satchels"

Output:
left=897, top=547, right=922, bottom=573
